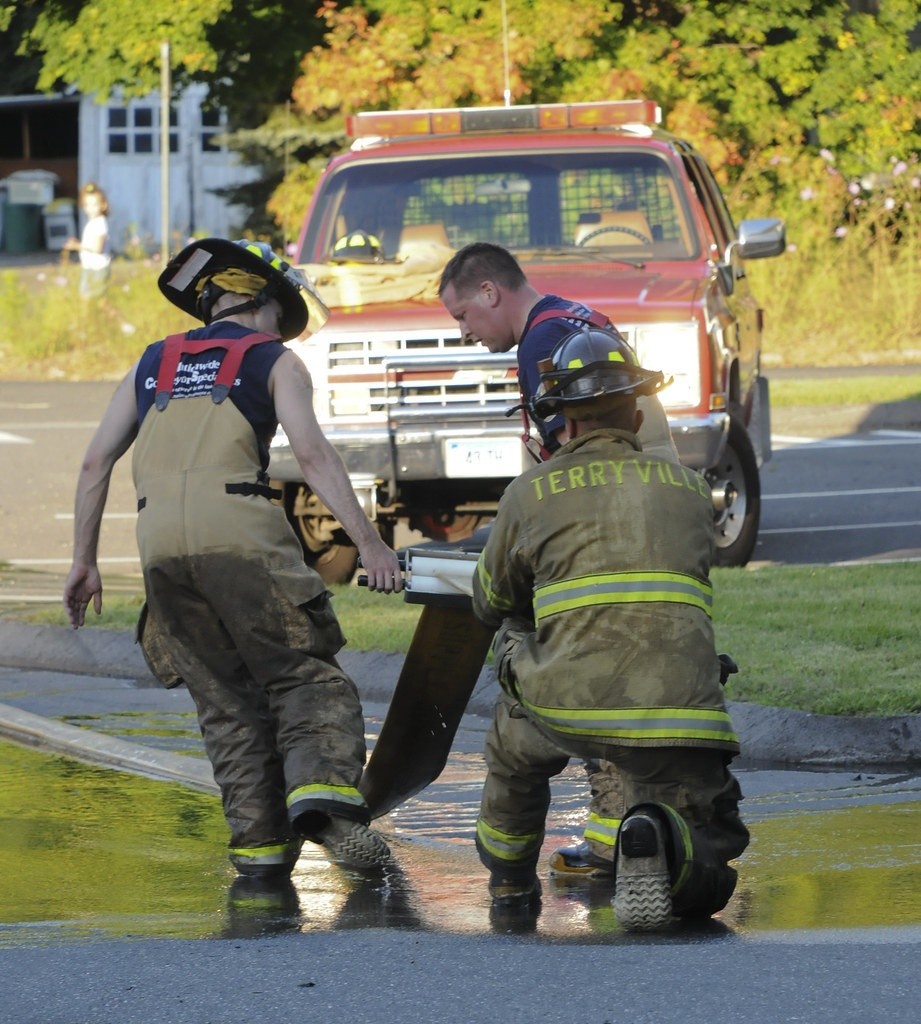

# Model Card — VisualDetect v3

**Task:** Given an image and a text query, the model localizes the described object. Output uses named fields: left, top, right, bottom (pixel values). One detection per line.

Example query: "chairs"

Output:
left=575, top=211, right=653, bottom=246
left=383, top=222, right=455, bottom=252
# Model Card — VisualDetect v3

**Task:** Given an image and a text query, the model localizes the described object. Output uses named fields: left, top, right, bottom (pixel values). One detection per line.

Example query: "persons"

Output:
left=438, top=241, right=685, bottom=875
left=64, top=237, right=406, bottom=876
left=473, top=332, right=752, bottom=929
left=62, top=185, right=138, bottom=335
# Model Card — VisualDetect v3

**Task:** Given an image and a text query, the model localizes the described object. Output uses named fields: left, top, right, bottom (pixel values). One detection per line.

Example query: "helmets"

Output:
left=505, top=326, right=674, bottom=419
left=157, top=238, right=309, bottom=342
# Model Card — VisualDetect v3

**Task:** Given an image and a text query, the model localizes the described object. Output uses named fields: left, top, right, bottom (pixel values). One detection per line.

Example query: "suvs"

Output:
left=230, top=98, right=789, bottom=592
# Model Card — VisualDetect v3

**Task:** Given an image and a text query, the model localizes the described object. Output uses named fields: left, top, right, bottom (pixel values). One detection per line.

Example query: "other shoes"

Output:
left=488, top=871, right=543, bottom=906
left=615, top=808, right=673, bottom=933
left=293, top=810, right=391, bottom=869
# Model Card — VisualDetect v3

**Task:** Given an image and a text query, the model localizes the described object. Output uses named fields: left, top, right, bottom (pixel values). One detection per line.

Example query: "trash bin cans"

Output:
left=42, top=197, right=78, bottom=253
left=3, top=202, right=43, bottom=254
left=7, top=171, right=55, bottom=206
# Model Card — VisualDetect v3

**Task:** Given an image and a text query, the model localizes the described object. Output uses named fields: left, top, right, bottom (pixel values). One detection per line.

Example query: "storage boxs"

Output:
left=6, top=176, right=53, bottom=205
left=44, top=215, right=76, bottom=250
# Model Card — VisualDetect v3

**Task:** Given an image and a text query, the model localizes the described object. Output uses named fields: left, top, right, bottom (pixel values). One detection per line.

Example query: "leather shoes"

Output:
left=549, top=841, right=615, bottom=877
